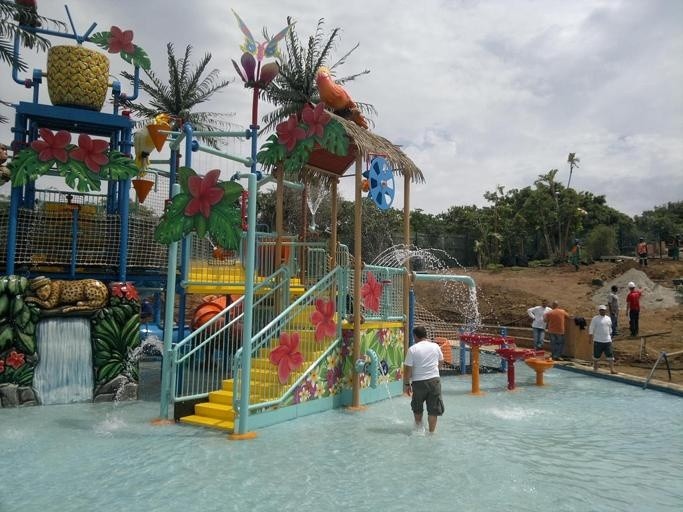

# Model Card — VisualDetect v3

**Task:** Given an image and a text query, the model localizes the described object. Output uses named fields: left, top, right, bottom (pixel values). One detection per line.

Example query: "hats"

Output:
left=628, top=282, right=635, bottom=288
left=598, top=305, right=607, bottom=311
left=640, top=237, right=643, bottom=240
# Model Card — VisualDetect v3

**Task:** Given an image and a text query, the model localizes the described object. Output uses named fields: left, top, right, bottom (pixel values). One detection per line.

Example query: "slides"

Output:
left=193, top=295, right=242, bottom=351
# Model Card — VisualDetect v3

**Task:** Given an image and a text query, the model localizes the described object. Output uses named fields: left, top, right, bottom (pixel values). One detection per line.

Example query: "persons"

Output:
left=572, top=239, right=582, bottom=272
left=635, top=237, right=649, bottom=267
left=608, top=285, right=620, bottom=335
left=589, top=305, right=619, bottom=374
left=625, top=282, right=649, bottom=336
left=527, top=299, right=570, bottom=361
left=403, top=326, right=444, bottom=434
